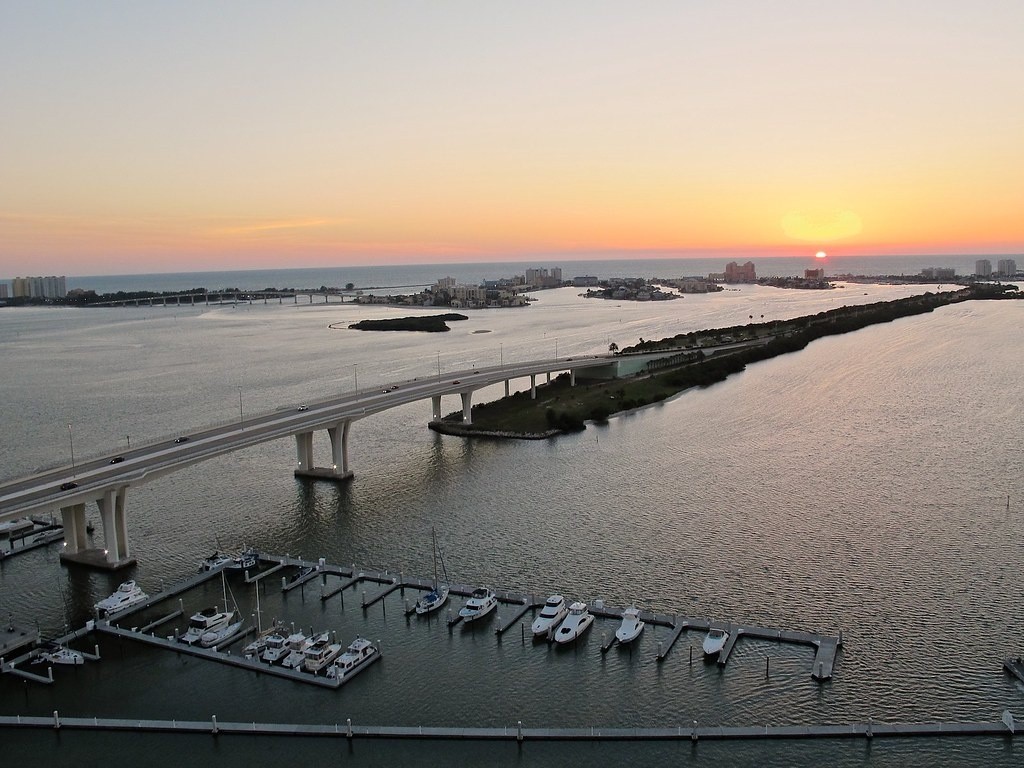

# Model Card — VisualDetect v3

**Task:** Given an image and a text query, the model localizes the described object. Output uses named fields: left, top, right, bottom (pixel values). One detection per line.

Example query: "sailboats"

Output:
left=181, top=570, right=235, bottom=642
left=415, top=526, right=452, bottom=615
left=39, top=577, right=85, bottom=667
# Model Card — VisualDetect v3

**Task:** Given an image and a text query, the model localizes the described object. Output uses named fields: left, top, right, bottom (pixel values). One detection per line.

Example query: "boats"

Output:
left=94, top=579, right=148, bottom=617
left=240, top=621, right=289, bottom=657
left=280, top=630, right=330, bottom=669
left=459, top=588, right=498, bottom=624
left=200, top=618, right=245, bottom=648
left=615, top=608, right=645, bottom=645
left=302, top=633, right=342, bottom=672
left=199, top=534, right=232, bottom=571
left=264, top=623, right=305, bottom=662
left=532, top=595, right=571, bottom=636
left=223, top=543, right=260, bottom=574
left=326, top=639, right=377, bottom=678
left=555, top=602, right=595, bottom=644
left=702, top=628, right=729, bottom=658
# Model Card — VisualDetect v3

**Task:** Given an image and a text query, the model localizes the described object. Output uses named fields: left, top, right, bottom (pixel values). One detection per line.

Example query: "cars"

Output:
left=298, top=404, right=309, bottom=411
left=110, top=457, right=124, bottom=464
left=61, top=482, right=77, bottom=491
left=382, top=385, right=399, bottom=394
left=174, top=436, right=191, bottom=444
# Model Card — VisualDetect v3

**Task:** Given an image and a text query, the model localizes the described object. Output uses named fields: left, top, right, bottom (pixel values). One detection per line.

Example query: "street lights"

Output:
left=68, top=425, right=76, bottom=478
left=237, top=385, right=244, bottom=431
left=500, top=343, right=503, bottom=371
left=555, top=337, right=557, bottom=363
left=438, top=350, right=441, bottom=383
left=354, top=363, right=358, bottom=402
